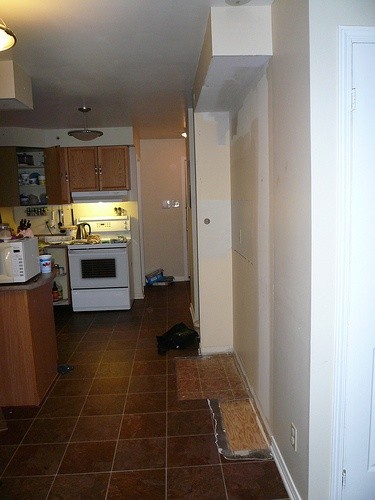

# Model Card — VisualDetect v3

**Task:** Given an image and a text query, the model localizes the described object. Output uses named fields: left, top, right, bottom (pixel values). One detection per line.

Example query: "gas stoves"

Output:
left=68, top=216, right=131, bottom=249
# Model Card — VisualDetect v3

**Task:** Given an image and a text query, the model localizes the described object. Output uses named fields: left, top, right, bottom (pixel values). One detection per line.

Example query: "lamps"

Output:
left=67, top=106, right=104, bottom=141
left=0, top=19, right=17, bottom=52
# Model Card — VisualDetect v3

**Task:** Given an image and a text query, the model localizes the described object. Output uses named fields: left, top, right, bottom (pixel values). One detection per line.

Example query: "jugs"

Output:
left=76, top=223, right=91, bottom=239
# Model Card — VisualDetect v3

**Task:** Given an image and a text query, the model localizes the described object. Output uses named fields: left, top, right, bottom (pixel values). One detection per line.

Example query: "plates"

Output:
left=48, top=242, right=61, bottom=245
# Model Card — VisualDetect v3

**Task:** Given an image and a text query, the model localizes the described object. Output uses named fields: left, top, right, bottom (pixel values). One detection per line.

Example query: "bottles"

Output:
left=52, top=281, right=58, bottom=302
left=58, top=285, right=63, bottom=301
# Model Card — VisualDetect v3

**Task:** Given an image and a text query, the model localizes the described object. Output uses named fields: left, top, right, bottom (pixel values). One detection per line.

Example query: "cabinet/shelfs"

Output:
left=37, top=233, right=71, bottom=307
left=0, top=144, right=135, bottom=208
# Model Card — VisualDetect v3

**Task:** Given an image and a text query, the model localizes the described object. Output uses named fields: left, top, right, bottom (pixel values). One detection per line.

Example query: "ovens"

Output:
left=68, top=248, right=134, bottom=312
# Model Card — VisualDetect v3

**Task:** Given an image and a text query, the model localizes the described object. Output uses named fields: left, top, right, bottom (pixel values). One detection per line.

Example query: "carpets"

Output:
left=207, top=396, right=271, bottom=461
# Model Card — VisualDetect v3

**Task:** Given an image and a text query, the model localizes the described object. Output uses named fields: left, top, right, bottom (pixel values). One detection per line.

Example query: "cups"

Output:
left=39, top=255, right=52, bottom=273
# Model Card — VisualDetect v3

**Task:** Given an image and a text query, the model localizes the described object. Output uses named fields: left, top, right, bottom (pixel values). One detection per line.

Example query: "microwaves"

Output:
left=0, top=237, right=41, bottom=283
